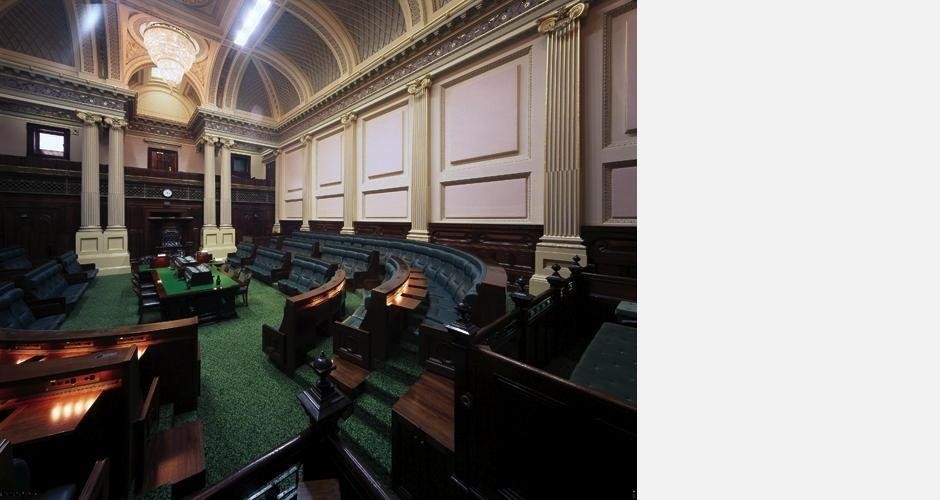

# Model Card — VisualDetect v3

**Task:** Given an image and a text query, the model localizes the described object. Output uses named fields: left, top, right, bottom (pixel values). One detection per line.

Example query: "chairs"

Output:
left=133, top=288, right=163, bottom=324
left=221, top=261, right=254, bottom=307
left=129, top=259, right=157, bottom=300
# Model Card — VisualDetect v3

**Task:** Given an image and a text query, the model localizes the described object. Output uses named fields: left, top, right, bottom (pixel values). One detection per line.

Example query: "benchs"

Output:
left=23, top=250, right=99, bottom=313
left=2, top=281, right=66, bottom=331
left=568, top=296, right=637, bottom=409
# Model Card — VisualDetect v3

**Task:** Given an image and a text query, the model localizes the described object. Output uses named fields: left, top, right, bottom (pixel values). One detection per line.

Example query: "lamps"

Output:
left=137, top=19, right=200, bottom=94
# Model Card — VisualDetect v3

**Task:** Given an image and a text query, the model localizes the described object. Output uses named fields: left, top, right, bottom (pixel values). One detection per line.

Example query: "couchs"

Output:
left=278, top=255, right=338, bottom=297
left=332, top=239, right=487, bottom=372
left=228, top=230, right=321, bottom=283
left=321, top=233, right=402, bottom=290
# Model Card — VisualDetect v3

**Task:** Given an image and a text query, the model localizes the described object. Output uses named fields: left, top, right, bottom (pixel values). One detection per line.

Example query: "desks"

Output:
left=151, top=264, right=239, bottom=328
left=1, top=316, right=199, bottom=498
